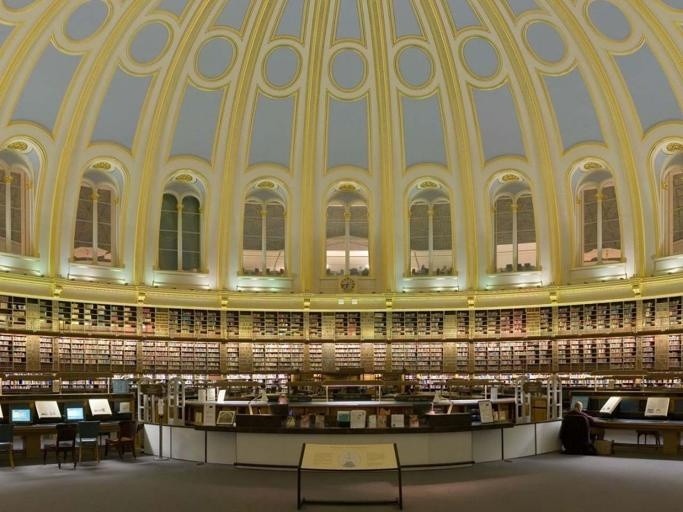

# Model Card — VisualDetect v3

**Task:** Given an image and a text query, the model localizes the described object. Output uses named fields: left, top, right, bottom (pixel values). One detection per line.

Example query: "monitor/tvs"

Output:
left=64, top=406, right=86, bottom=422
left=10, top=408, right=33, bottom=424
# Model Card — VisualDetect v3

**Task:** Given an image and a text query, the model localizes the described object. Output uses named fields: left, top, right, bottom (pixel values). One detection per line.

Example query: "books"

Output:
left=1, top=292, right=682, bottom=427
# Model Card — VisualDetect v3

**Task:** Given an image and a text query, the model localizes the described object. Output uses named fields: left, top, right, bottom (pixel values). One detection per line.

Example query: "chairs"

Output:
left=54, top=419, right=137, bottom=467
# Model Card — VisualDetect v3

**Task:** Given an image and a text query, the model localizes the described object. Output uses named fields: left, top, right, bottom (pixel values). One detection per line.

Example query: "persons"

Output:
left=565, top=401, right=606, bottom=443
